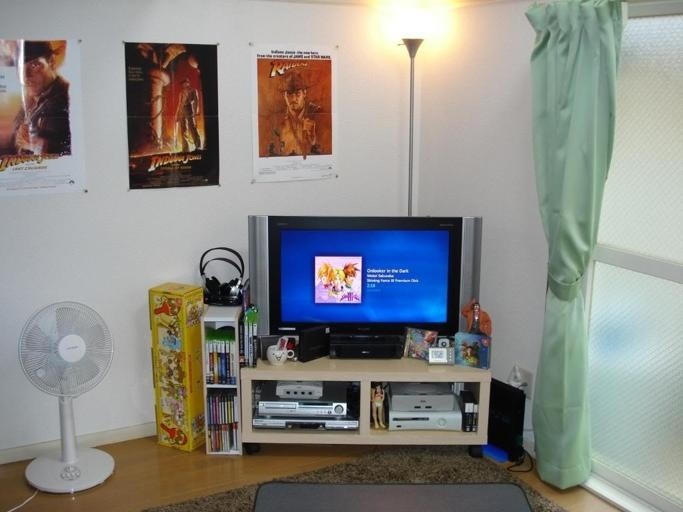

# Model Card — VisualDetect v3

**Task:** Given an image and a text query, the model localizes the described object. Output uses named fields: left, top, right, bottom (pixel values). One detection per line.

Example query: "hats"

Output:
left=6, top=41, right=62, bottom=67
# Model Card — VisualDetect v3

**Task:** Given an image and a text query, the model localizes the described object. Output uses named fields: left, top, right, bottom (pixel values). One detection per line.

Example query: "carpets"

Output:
left=136, top=444, right=571, bottom=510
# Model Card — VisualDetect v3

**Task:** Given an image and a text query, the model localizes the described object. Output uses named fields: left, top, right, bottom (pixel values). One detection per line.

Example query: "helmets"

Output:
left=278, top=71, right=309, bottom=92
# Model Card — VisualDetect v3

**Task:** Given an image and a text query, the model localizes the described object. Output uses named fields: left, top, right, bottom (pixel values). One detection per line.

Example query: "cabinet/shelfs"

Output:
left=200, top=306, right=240, bottom=455
left=239, top=354, right=490, bottom=457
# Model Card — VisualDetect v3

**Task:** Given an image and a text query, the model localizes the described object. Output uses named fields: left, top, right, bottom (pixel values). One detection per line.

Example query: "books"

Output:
left=450, top=381, right=478, bottom=433
left=204, top=325, right=240, bottom=453
left=237, top=311, right=259, bottom=370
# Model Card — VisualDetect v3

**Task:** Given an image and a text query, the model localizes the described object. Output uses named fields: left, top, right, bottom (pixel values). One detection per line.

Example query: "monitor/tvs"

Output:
left=247, top=213, right=483, bottom=340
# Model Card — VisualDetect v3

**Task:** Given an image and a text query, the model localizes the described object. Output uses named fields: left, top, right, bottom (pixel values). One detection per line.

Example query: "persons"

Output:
left=173, top=77, right=202, bottom=153
left=2, top=40, right=71, bottom=159
left=368, top=382, right=386, bottom=430
left=461, top=297, right=492, bottom=337
left=263, top=71, right=329, bottom=156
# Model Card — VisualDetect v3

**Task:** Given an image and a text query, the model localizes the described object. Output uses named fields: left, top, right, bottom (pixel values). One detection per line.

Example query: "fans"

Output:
left=17, top=300, right=114, bottom=494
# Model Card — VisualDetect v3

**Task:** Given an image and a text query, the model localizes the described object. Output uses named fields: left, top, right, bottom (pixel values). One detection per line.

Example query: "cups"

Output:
left=266, top=345, right=294, bottom=366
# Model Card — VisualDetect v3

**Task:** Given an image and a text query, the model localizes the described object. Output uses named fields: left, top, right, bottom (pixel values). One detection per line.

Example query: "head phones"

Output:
left=198, top=247, right=246, bottom=305
